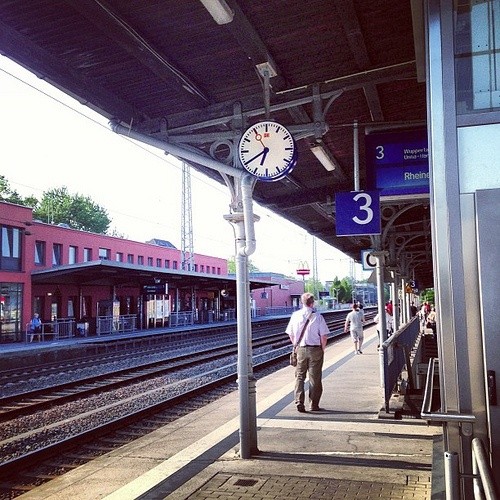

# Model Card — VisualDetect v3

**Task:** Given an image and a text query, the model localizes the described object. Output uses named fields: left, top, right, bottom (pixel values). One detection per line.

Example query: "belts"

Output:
left=304, top=345, right=320, bottom=348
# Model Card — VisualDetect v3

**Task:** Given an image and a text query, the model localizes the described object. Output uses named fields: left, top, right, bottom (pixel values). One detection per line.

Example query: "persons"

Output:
left=284, top=292, right=330, bottom=413
left=27, top=313, right=42, bottom=342
left=343, top=304, right=366, bottom=355
left=357, top=299, right=435, bottom=348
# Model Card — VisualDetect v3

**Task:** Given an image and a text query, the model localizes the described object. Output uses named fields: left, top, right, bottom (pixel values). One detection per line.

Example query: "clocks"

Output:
left=238, top=120, right=299, bottom=183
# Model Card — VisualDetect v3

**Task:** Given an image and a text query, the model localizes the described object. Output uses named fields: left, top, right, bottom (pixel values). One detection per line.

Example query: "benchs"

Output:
left=26, top=333, right=57, bottom=343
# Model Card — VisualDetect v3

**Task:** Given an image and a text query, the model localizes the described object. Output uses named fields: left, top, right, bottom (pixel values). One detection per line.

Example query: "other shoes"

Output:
left=309, top=406, right=319, bottom=411
left=297, top=401, right=305, bottom=413
left=355, top=352, right=358, bottom=354
left=358, top=350, right=362, bottom=354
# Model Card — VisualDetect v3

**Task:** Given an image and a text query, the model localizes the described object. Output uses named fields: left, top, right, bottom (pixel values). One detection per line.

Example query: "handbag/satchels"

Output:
left=290, top=352, right=297, bottom=367
